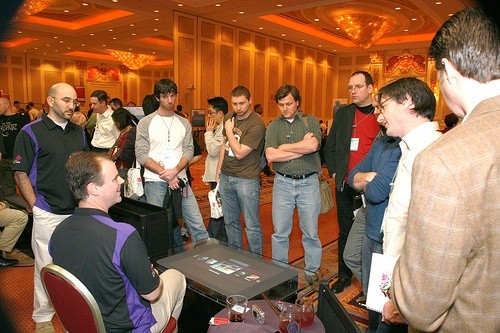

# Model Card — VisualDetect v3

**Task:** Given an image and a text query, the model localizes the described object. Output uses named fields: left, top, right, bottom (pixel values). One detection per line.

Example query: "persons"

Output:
left=253, top=104, right=275, bottom=176
left=264, top=85, right=322, bottom=292
left=135, top=78, right=209, bottom=242
left=319, top=119, right=326, bottom=127
left=325, top=70, right=382, bottom=293
left=12, top=82, right=91, bottom=333
left=49, top=151, right=186, bottom=333
left=343, top=76, right=443, bottom=333
left=202, top=96, right=229, bottom=247
left=216, top=86, right=266, bottom=258
left=0, top=90, right=203, bottom=267
left=439, top=113, right=459, bottom=134
left=382, top=6, right=500, bottom=333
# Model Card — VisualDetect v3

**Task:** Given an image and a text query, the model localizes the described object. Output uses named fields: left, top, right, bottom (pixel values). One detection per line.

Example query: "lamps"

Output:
left=302, top=1, right=424, bottom=50
left=109, top=46, right=161, bottom=70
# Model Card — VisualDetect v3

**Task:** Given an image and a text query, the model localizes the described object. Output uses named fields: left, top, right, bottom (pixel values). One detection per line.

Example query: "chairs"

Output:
left=40, top=264, right=178, bottom=333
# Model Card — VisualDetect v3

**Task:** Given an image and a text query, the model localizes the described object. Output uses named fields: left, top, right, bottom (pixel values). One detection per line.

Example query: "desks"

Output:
left=150, top=238, right=319, bottom=304
left=208, top=300, right=325, bottom=333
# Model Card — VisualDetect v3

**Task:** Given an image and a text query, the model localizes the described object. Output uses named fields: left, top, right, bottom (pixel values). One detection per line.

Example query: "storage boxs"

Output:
left=252, top=304, right=266, bottom=324
left=108, top=195, right=169, bottom=260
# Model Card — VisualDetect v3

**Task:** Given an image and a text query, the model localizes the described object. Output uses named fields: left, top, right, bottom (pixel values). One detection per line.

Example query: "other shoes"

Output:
left=182, top=234, right=189, bottom=243
left=35, top=321, right=54, bottom=333
left=305, top=274, right=320, bottom=292
left=357, top=300, right=366, bottom=309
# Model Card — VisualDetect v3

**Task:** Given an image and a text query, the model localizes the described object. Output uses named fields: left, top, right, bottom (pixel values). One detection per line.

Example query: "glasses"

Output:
left=208, top=111, right=217, bottom=115
left=52, top=97, right=80, bottom=107
left=376, top=96, right=395, bottom=114
left=346, top=85, right=368, bottom=91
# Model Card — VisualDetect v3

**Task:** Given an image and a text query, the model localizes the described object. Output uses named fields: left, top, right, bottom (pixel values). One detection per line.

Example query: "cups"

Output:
left=226, top=294, right=248, bottom=323
left=279, top=305, right=301, bottom=333
left=294, top=298, right=314, bottom=328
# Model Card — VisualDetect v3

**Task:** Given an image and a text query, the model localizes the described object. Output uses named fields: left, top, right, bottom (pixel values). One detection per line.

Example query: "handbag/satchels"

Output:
left=208, top=183, right=223, bottom=219
left=320, top=179, right=334, bottom=214
left=123, top=168, right=144, bottom=200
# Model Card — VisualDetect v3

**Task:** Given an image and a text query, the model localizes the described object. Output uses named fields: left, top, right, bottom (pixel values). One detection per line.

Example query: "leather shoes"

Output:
left=331, top=276, right=351, bottom=294
left=0, top=255, right=19, bottom=268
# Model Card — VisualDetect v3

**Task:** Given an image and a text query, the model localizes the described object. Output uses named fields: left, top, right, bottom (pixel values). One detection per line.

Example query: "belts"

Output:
left=276, top=171, right=315, bottom=180
